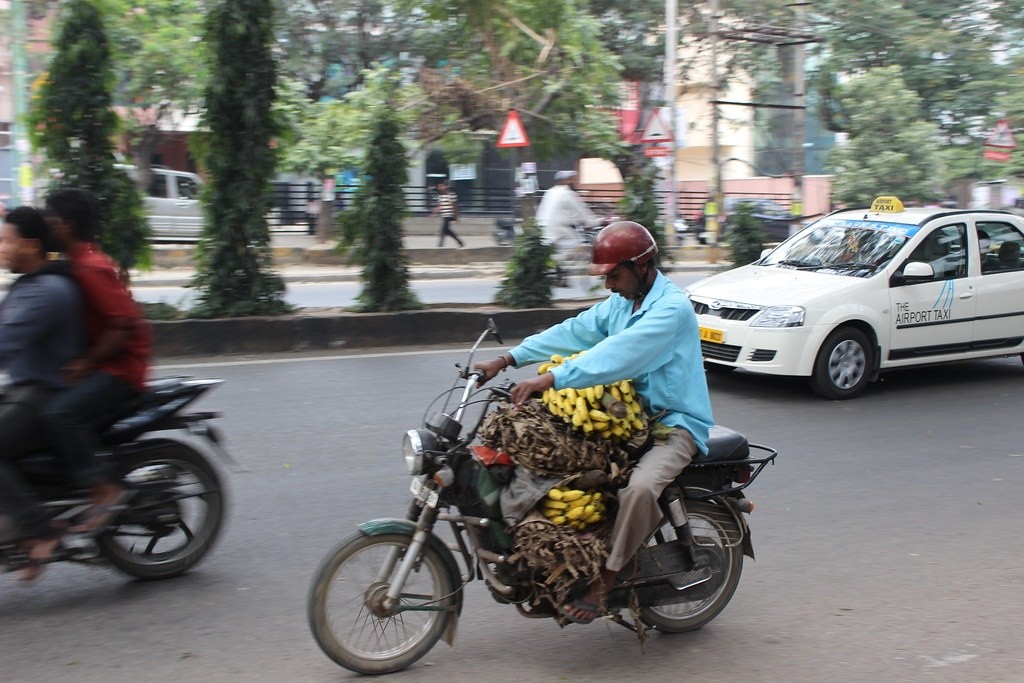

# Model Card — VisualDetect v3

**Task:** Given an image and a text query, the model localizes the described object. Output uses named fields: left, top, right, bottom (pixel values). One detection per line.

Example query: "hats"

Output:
left=554, top=171, right=578, bottom=180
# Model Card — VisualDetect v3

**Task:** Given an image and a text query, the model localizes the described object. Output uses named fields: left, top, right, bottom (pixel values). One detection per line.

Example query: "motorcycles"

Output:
left=308, top=316, right=778, bottom=675
left=1, top=375, right=236, bottom=579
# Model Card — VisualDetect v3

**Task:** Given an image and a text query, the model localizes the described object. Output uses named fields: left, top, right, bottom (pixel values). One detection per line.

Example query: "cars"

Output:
left=681, top=197, right=1024, bottom=401
left=697, top=196, right=792, bottom=245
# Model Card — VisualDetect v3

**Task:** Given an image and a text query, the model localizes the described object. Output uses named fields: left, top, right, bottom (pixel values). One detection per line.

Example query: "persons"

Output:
left=0, top=188, right=153, bottom=564
left=436, top=181, right=465, bottom=249
left=474, top=222, right=713, bottom=623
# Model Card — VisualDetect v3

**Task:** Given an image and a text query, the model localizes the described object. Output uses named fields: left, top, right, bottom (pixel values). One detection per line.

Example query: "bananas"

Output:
left=536, top=350, right=646, bottom=441
left=541, top=486, right=607, bottom=531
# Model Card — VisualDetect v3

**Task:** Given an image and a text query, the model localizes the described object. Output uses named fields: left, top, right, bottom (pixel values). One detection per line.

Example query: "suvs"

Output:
left=112, top=167, right=208, bottom=243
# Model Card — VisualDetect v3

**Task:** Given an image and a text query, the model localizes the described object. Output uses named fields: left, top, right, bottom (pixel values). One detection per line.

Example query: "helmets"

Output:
left=586, top=221, right=658, bottom=275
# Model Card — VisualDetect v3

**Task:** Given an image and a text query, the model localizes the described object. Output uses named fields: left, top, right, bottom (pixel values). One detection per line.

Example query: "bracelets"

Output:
left=498, top=355, right=508, bottom=372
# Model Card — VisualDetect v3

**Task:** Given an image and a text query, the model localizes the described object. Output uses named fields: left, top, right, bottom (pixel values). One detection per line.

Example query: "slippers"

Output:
left=562, top=594, right=617, bottom=624
left=77, top=484, right=138, bottom=537
